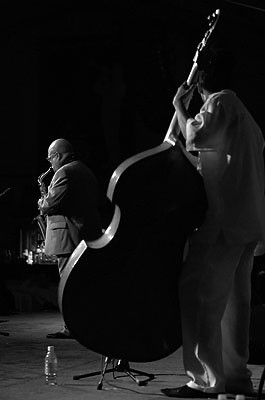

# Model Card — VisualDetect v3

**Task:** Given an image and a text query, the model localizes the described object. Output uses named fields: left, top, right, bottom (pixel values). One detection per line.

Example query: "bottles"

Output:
left=44, top=346, right=57, bottom=386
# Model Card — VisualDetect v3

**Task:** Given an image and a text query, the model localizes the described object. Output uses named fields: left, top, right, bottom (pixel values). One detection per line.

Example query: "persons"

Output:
left=36, top=138, right=107, bottom=341
left=159, top=64, right=265, bottom=399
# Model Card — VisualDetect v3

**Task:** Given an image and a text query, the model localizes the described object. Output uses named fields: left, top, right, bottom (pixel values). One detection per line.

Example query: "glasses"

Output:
left=46, top=152, right=58, bottom=161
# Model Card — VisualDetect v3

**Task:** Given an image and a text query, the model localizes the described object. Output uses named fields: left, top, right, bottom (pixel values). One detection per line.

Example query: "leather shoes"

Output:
left=160, top=385, right=226, bottom=399
left=47, top=332, right=74, bottom=339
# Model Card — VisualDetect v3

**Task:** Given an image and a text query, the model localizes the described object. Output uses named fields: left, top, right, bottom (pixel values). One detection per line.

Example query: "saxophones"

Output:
left=23, top=165, right=59, bottom=266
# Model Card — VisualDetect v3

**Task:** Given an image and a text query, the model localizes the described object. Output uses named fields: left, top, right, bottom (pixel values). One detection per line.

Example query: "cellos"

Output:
left=54, top=6, right=237, bottom=393
